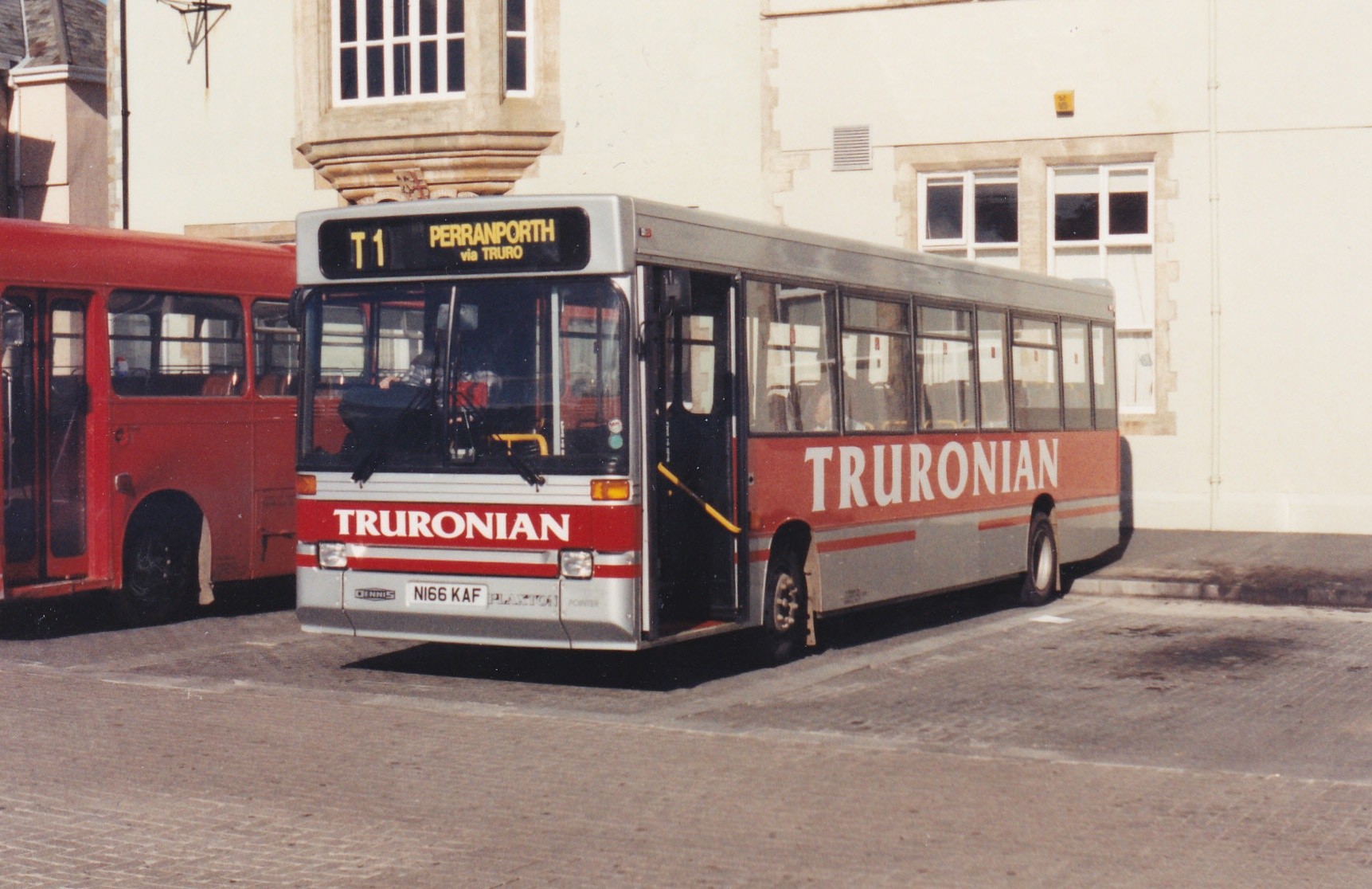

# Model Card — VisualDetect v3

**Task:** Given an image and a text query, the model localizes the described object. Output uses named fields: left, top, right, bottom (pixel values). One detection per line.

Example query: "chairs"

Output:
left=768, top=379, right=1109, bottom=431
left=113, top=368, right=351, bottom=394
left=452, top=365, right=504, bottom=406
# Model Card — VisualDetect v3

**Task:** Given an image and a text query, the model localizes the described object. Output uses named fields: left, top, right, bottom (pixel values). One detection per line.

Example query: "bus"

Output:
left=0, top=214, right=617, bottom=626
left=288, top=194, right=1118, bottom=670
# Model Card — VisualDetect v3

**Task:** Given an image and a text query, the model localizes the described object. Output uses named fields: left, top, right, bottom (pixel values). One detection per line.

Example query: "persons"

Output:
left=813, top=389, right=870, bottom=431
left=379, top=314, right=504, bottom=464
